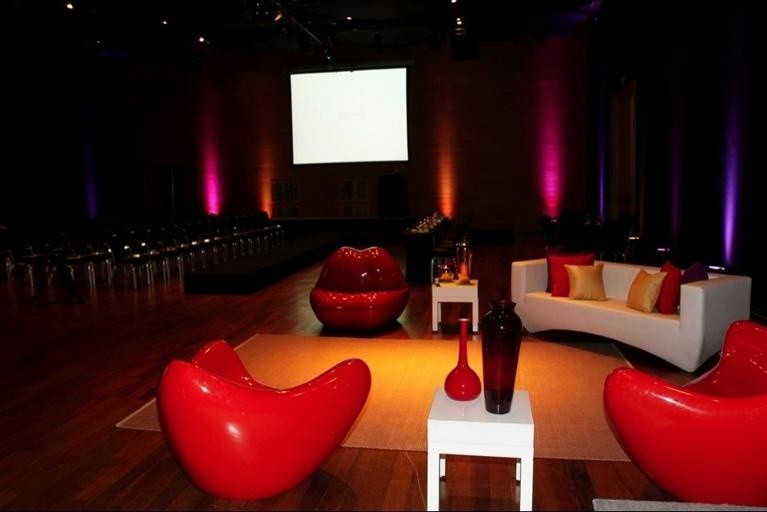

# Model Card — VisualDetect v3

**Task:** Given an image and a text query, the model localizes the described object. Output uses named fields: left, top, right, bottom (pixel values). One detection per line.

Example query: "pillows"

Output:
left=546, top=252, right=595, bottom=297
left=564, top=263, right=609, bottom=301
left=627, top=267, right=668, bottom=313
left=680, top=261, right=708, bottom=284
left=654, top=259, right=681, bottom=315
left=545, top=246, right=596, bottom=293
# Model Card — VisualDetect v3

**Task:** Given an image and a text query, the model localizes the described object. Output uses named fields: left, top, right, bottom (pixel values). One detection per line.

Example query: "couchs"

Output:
left=510, top=257, right=752, bottom=375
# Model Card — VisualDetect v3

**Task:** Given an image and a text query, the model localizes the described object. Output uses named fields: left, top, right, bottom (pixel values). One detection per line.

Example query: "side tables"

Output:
left=431, top=279, right=479, bottom=335
left=426, top=388, right=535, bottom=512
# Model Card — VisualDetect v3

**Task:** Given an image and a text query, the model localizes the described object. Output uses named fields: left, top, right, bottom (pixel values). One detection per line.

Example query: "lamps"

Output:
left=240, top=2, right=338, bottom=67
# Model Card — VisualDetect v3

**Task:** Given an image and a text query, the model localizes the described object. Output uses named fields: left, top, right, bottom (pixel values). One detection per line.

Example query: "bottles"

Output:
left=446, top=315, right=482, bottom=402
left=478, top=302, right=524, bottom=415
left=456, top=230, right=474, bottom=281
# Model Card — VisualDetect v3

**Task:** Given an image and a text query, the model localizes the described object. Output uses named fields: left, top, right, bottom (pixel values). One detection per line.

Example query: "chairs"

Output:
left=602, top=320, right=767, bottom=507
left=156, top=336, right=372, bottom=502
left=0, top=208, right=287, bottom=310
left=309, top=246, right=412, bottom=330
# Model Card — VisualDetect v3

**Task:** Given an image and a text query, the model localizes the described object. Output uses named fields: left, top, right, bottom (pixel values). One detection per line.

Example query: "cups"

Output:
left=437, top=255, right=455, bottom=281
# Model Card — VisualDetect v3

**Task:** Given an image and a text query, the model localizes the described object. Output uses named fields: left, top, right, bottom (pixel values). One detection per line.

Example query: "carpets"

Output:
left=114, top=330, right=644, bottom=463
